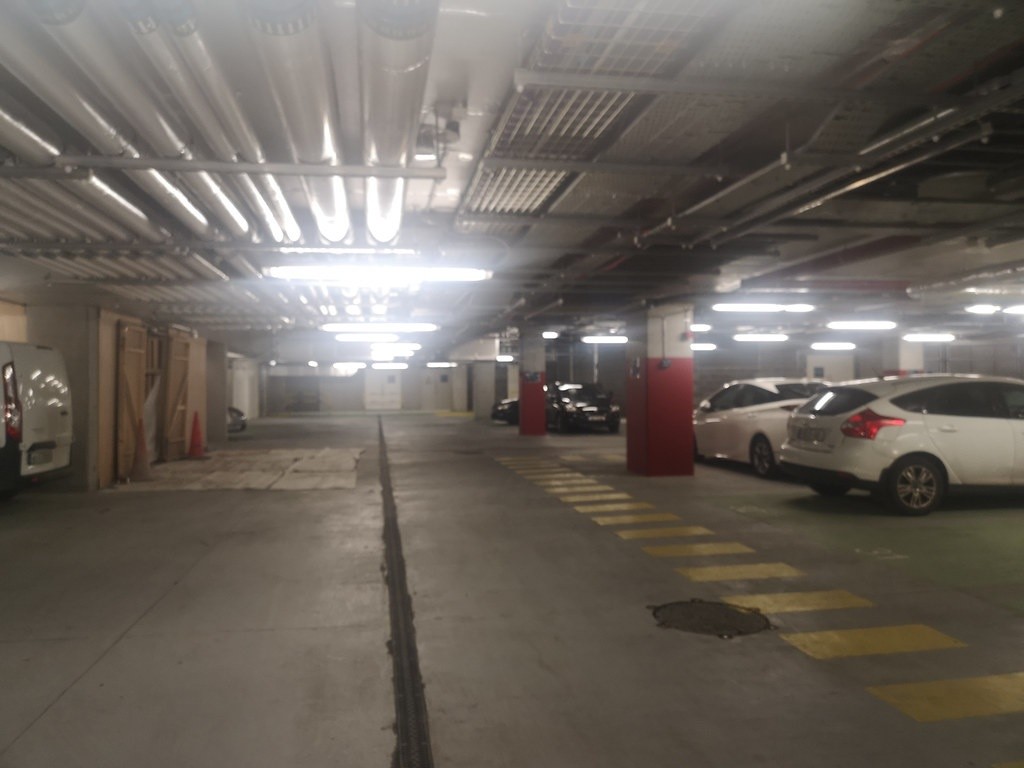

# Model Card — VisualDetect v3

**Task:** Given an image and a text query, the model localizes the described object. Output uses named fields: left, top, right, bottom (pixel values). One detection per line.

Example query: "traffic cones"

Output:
left=124, top=418, right=155, bottom=479
left=179, top=411, right=211, bottom=461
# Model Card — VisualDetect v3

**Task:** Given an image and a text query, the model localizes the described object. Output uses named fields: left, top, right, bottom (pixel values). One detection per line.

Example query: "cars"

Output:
left=692, top=376, right=830, bottom=478
left=491, top=399, right=520, bottom=424
left=779, top=371, right=1024, bottom=516
left=228, top=405, right=246, bottom=432
left=545, top=381, right=620, bottom=436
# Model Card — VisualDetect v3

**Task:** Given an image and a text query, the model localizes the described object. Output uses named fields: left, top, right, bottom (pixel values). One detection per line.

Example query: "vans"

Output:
left=0, top=341, right=71, bottom=497
